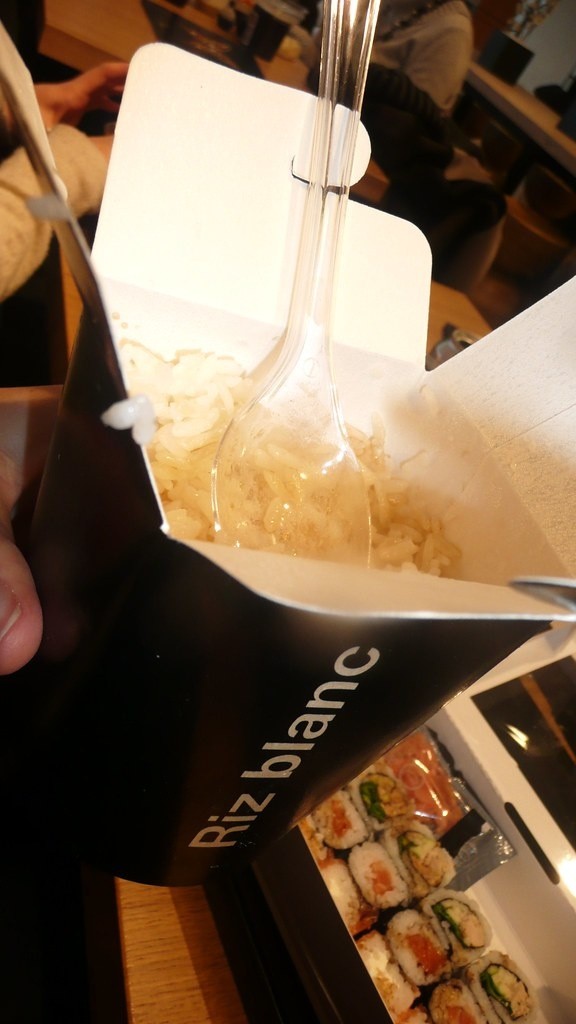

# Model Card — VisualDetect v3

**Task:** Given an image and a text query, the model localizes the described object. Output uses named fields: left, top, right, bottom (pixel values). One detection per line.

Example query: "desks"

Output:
left=36, top=0, right=390, bottom=208
left=54, top=217, right=251, bottom=1024
left=462, top=43, right=576, bottom=178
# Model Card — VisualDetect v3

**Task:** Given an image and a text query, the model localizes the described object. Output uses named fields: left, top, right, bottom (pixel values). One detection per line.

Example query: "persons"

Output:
left=311, top=0, right=474, bottom=160
left=0, top=386, right=66, bottom=671
left=0, top=0, right=131, bottom=302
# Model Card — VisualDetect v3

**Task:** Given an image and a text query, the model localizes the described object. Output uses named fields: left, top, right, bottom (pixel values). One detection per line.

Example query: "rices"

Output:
left=99, top=335, right=464, bottom=579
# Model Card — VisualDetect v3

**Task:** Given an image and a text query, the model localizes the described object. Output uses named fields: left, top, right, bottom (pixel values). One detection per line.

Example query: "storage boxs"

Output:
left=0, top=28, right=576, bottom=881
left=249, top=624, right=576, bottom=1024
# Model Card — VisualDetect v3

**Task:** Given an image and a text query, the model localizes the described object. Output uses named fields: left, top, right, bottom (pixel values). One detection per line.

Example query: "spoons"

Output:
left=210, top=1, right=380, bottom=565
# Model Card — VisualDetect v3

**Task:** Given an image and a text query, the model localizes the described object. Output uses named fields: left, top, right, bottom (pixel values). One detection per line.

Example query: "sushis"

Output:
left=295, top=758, right=543, bottom=1024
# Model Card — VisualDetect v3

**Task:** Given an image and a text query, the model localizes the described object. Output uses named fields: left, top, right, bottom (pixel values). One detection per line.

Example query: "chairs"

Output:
left=422, top=102, right=575, bottom=370
